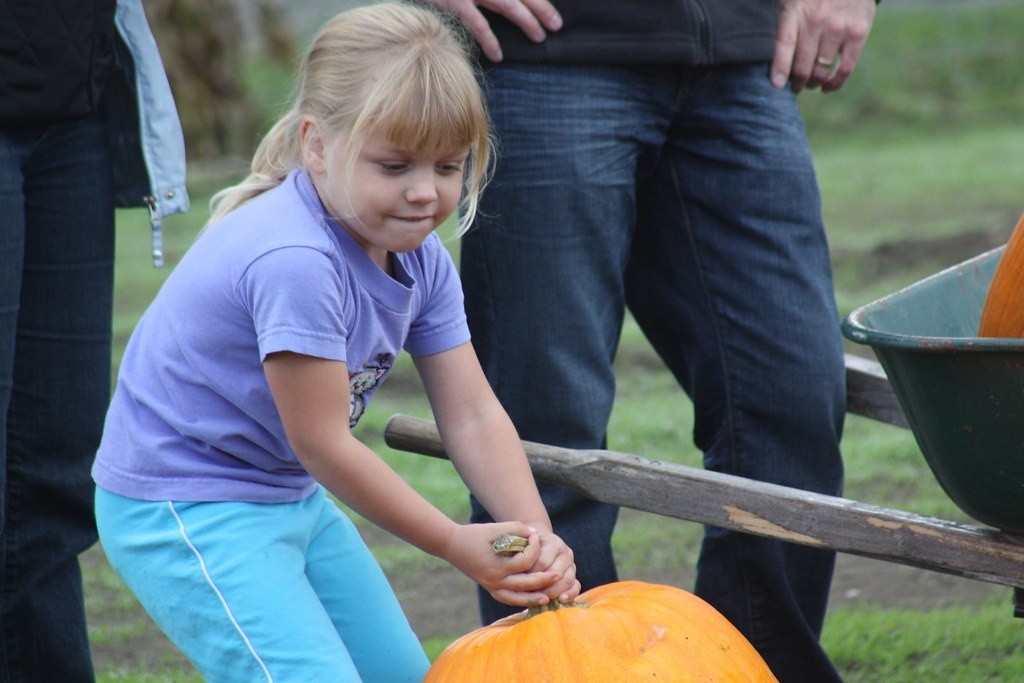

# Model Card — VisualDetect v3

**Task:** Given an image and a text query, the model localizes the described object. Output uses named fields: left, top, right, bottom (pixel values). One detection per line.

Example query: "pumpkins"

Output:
left=421, top=535, right=783, bottom=682
left=978, top=210, right=1024, bottom=339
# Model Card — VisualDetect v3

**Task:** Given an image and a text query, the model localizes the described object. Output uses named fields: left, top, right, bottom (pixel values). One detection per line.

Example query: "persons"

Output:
left=2, top=0, right=193, bottom=683
left=436, top=1, right=884, bottom=681
left=89, top=10, right=582, bottom=683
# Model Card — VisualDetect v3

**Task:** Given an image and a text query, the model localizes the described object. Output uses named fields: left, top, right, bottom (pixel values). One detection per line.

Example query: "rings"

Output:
left=816, top=55, right=833, bottom=68
